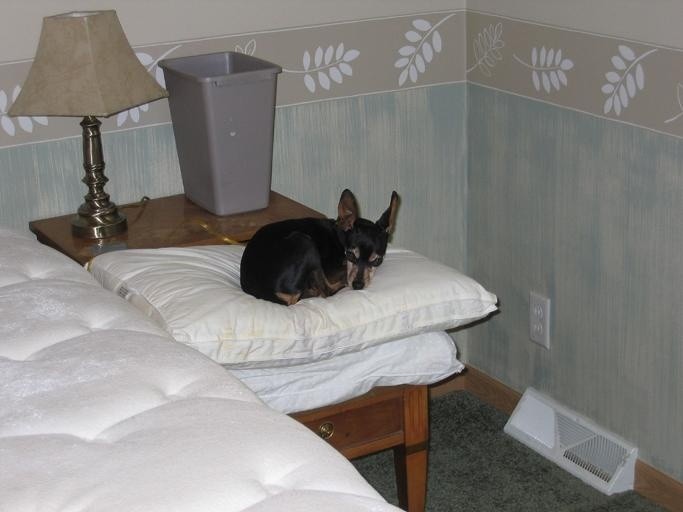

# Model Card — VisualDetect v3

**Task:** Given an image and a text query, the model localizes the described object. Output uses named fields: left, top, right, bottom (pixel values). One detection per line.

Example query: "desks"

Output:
left=29, top=184, right=329, bottom=273
left=286, top=384, right=428, bottom=511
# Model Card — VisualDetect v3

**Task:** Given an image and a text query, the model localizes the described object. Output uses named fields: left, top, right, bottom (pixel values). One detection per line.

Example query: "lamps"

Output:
left=5, top=9, right=169, bottom=239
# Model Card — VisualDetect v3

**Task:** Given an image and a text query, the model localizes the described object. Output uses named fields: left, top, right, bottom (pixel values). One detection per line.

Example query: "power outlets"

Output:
left=528, top=291, right=551, bottom=351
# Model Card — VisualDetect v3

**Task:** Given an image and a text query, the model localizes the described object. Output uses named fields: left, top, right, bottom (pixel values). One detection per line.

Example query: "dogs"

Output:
left=239, top=189, right=400, bottom=307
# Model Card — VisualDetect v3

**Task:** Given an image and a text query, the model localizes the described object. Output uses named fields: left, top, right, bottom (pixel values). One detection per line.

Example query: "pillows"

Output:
left=225, top=331, right=465, bottom=413
left=87, top=246, right=499, bottom=368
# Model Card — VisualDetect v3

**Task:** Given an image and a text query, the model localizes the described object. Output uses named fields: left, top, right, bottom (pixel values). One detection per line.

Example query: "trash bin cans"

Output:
left=157, top=50, right=283, bottom=218
